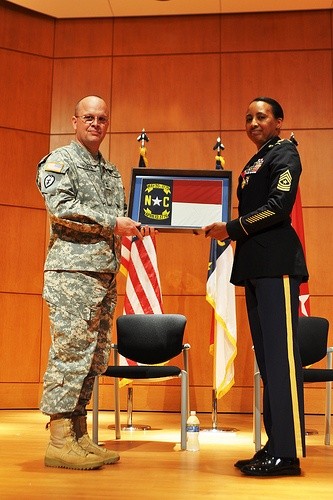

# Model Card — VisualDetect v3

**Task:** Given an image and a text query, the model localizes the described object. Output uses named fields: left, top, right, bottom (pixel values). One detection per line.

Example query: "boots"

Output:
left=44, top=413, right=120, bottom=471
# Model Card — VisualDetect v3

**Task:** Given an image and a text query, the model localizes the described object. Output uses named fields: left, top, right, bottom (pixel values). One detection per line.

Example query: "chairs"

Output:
left=92, top=314, right=191, bottom=449
left=251, top=316, right=333, bottom=453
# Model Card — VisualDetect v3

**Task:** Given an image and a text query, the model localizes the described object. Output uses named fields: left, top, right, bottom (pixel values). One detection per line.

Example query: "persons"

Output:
left=36, top=96, right=158, bottom=470
left=202, top=97, right=309, bottom=477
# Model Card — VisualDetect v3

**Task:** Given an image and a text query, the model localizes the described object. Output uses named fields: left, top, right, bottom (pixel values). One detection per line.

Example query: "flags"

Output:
left=119, top=147, right=181, bottom=389
left=290, top=183, right=310, bottom=369
left=205, top=156, right=237, bottom=399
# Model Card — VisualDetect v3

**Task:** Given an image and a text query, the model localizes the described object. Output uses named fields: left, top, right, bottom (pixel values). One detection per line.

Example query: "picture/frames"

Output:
left=127, top=167, right=232, bottom=234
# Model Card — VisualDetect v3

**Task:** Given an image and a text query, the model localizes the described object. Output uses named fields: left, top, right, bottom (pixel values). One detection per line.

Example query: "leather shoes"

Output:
left=234, top=447, right=301, bottom=476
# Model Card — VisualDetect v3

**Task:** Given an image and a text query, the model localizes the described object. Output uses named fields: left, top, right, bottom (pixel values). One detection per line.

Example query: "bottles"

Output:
left=186, top=411, right=200, bottom=451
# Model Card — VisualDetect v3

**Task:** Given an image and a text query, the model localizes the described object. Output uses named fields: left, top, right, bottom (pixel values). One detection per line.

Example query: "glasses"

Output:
left=74, top=115, right=109, bottom=124
